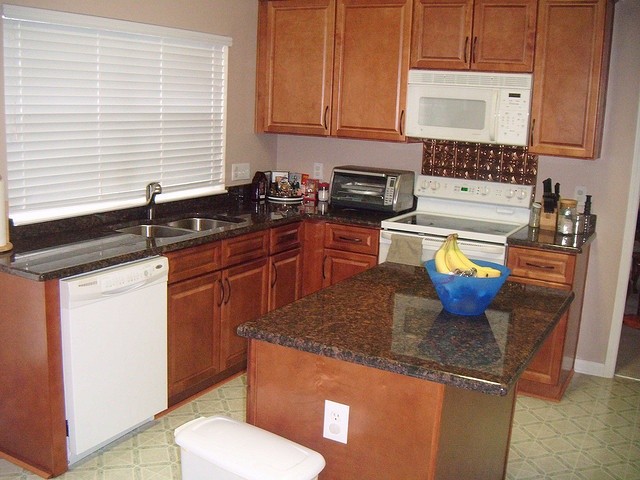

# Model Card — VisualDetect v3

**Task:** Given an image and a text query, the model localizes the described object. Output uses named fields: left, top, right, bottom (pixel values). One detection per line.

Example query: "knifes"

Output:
left=542, top=177, right=554, bottom=212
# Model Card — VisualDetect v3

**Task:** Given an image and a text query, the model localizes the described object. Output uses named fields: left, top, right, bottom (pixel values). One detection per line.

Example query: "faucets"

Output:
left=144, top=180, right=164, bottom=218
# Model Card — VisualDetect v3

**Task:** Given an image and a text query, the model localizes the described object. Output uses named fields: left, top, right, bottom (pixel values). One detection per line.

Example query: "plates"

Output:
left=345, top=183, right=385, bottom=196
left=266, top=195, right=304, bottom=203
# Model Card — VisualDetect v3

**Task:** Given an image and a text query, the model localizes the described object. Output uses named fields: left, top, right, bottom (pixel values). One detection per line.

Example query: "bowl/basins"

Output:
left=423, top=258, right=511, bottom=318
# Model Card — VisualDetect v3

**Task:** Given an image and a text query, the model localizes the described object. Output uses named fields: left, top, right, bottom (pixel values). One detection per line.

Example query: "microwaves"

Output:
left=403, top=68, right=533, bottom=147
left=381, top=211, right=522, bottom=245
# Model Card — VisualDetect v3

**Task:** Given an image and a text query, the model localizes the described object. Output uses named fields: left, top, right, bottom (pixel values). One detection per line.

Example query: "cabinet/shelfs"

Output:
left=255, top=1, right=337, bottom=137
left=529, top=1, right=614, bottom=161
left=321, top=222, right=380, bottom=289
left=507, top=246, right=593, bottom=404
left=269, top=222, right=304, bottom=313
left=161, top=230, right=270, bottom=411
left=410, top=1, right=538, bottom=73
left=331, top=1, right=413, bottom=142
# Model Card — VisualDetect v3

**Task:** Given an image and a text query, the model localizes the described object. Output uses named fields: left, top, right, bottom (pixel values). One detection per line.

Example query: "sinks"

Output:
left=169, top=215, right=243, bottom=233
left=113, top=224, right=196, bottom=247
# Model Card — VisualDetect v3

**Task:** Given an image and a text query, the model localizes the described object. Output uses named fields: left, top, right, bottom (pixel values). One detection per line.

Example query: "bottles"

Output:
left=530, top=203, right=541, bottom=228
left=318, top=183, right=330, bottom=201
left=573, top=215, right=585, bottom=235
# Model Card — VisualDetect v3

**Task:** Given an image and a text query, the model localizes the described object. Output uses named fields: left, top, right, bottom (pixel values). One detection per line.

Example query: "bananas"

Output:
left=434, top=234, right=502, bottom=278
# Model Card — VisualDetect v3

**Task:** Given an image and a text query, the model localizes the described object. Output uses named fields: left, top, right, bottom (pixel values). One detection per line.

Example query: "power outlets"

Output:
left=321, top=399, right=350, bottom=444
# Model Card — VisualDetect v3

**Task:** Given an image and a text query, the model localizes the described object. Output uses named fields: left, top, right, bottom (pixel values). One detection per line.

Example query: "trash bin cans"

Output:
left=175, top=415, right=326, bottom=480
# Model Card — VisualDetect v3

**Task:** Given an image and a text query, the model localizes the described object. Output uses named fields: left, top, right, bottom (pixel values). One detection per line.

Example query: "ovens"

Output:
left=378, top=230, right=506, bottom=268
left=329, top=164, right=415, bottom=213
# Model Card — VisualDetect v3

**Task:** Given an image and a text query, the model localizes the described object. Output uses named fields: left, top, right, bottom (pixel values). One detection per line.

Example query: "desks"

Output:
left=232, top=260, right=576, bottom=479
